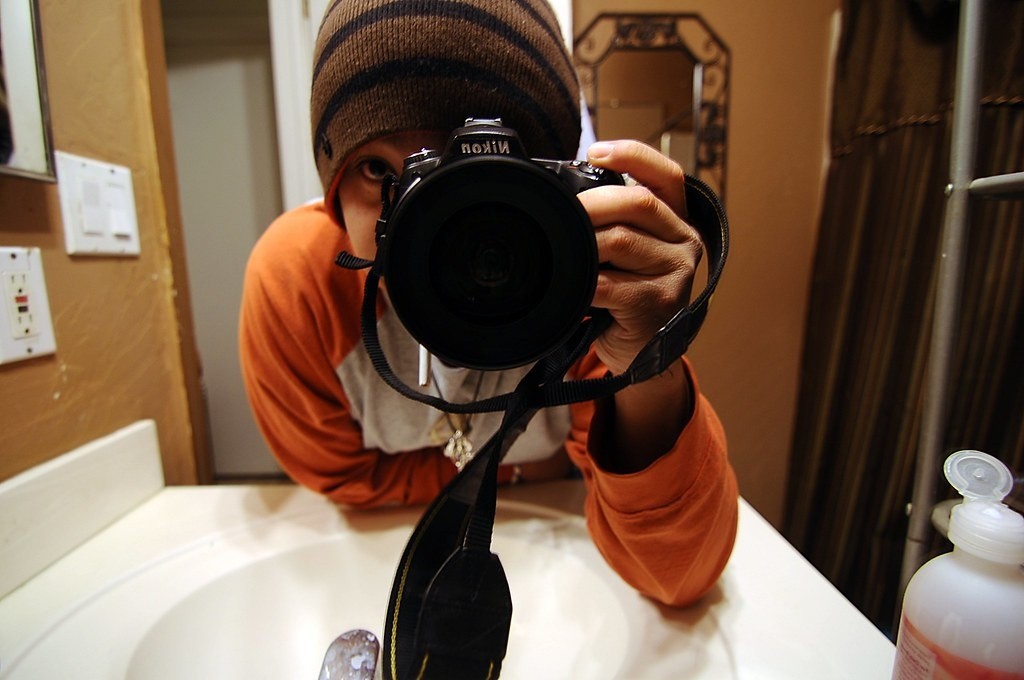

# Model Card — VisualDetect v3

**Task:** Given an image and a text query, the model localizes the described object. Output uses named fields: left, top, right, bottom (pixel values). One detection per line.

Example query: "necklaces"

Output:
left=431, top=367, right=485, bottom=472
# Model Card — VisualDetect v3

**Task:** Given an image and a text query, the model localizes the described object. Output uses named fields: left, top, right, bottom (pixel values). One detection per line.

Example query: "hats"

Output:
left=310, top=0, right=582, bottom=231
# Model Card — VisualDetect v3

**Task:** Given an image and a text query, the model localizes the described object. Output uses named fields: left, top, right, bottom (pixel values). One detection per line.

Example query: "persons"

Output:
left=237, top=0, right=738, bottom=609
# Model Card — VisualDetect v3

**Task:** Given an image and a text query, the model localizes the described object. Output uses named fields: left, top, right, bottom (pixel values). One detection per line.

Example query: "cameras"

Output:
left=381, top=117, right=627, bottom=372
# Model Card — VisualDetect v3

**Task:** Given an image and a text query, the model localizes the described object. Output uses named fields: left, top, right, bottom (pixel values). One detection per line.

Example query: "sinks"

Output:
left=2, top=496, right=741, bottom=680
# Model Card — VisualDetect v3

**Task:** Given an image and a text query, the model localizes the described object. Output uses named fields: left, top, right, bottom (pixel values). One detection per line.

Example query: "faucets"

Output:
left=318, top=629, right=379, bottom=680
